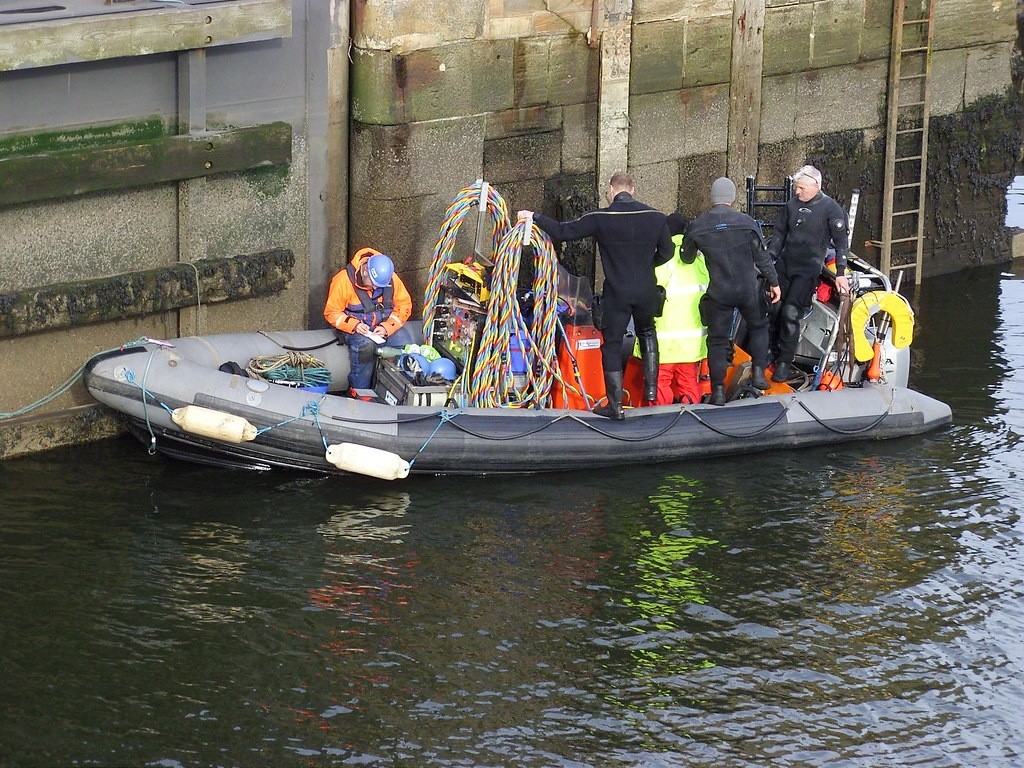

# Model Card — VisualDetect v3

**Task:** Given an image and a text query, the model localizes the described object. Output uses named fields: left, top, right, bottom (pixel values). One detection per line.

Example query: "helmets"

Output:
left=400, top=353, right=456, bottom=380
left=368, top=254, right=394, bottom=287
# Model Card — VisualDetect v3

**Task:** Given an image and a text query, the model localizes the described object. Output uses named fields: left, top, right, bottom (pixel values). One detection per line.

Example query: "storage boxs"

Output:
left=373, top=356, right=461, bottom=408
left=296, top=383, right=329, bottom=394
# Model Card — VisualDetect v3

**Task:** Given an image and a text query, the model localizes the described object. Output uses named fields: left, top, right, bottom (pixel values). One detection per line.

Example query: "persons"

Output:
left=632, top=211, right=711, bottom=405
left=765, top=165, right=850, bottom=382
left=324, top=248, right=412, bottom=400
left=679, top=177, right=782, bottom=405
left=517, top=173, right=674, bottom=420
left=522, top=269, right=592, bottom=320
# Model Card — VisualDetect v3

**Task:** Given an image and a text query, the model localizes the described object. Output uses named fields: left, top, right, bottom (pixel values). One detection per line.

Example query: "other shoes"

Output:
left=772, top=360, right=791, bottom=382
left=751, top=365, right=770, bottom=390
left=708, top=384, right=726, bottom=406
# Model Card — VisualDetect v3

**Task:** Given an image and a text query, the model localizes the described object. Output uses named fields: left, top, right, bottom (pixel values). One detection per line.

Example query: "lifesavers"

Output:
left=844, top=290, right=914, bottom=363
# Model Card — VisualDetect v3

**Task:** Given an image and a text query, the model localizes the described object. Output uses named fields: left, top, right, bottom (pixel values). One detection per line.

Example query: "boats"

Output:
left=82, top=318, right=954, bottom=480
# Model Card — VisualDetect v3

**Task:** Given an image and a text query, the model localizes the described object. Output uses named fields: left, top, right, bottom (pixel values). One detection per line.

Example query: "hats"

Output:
left=710, top=176, right=736, bottom=205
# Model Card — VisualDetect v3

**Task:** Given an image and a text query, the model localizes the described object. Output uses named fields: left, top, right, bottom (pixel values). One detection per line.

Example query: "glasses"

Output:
left=797, top=170, right=818, bottom=184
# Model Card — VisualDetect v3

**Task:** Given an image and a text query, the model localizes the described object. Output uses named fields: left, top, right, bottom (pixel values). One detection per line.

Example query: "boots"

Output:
left=592, top=371, right=625, bottom=420
left=642, top=351, right=659, bottom=401
